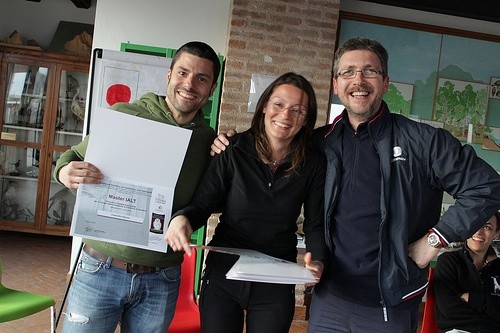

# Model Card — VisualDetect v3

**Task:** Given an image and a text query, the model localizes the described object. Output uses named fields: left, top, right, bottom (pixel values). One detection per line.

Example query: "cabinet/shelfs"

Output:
left=121, top=43, right=223, bottom=296
left=0, top=46, right=91, bottom=236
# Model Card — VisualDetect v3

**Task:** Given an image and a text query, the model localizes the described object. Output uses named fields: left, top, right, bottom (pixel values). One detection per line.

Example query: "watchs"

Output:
left=428, top=229, right=445, bottom=248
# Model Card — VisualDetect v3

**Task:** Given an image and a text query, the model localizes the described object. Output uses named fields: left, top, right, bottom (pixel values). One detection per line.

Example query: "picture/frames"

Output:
left=432, top=77, right=490, bottom=145
left=381, top=82, right=415, bottom=118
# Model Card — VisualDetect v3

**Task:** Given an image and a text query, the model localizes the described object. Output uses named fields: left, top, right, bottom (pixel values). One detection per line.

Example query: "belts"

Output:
left=83, top=245, right=154, bottom=275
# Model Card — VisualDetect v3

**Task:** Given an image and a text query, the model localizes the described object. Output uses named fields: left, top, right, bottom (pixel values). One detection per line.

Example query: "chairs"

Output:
left=0, top=252, right=56, bottom=333
left=166, top=242, right=202, bottom=333
left=421, top=269, right=441, bottom=333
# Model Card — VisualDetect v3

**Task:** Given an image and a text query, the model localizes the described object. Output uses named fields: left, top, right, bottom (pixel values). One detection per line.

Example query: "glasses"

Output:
left=267, top=100, right=308, bottom=118
left=335, top=69, right=386, bottom=79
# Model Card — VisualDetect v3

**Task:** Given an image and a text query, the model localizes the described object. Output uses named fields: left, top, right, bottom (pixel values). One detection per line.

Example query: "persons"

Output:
left=166, top=72, right=326, bottom=333
left=210, top=37, right=500, bottom=333
left=53, top=42, right=223, bottom=333
left=434, top=209, right=500, bottom=333
